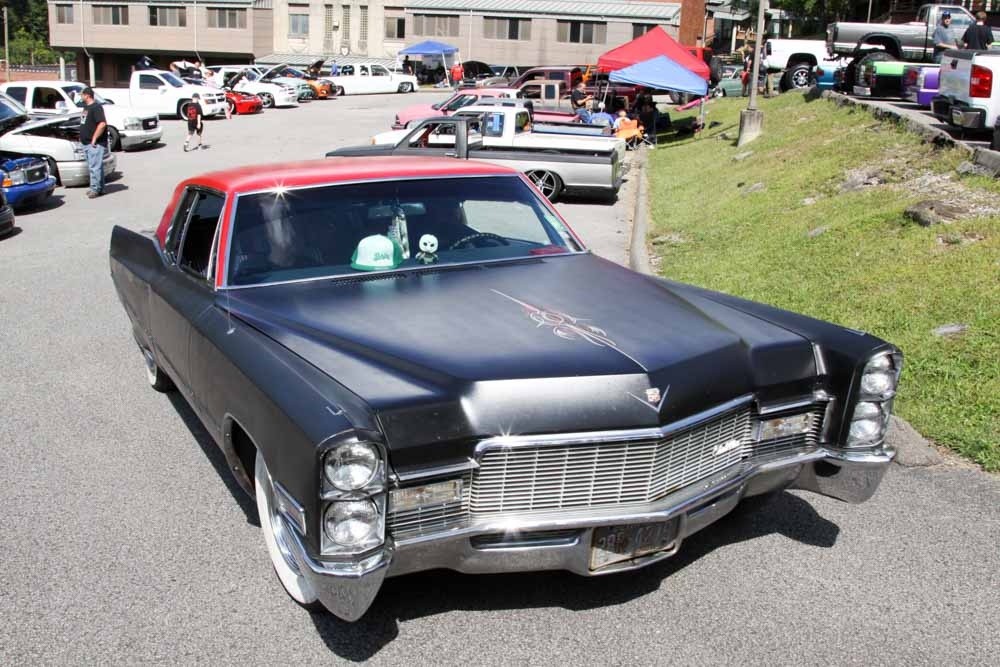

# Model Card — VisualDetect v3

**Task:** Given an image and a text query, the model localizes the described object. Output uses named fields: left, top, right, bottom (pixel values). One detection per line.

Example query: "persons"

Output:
left=183, top=93, right=207, bottom=152
left=571, top=82, right=658, bottom=150
left=402, top=55, right=445, bottom=83
left=169, top=61, right=217, bottom=88
left=330, top=60, right=338, bottom=77
left=81, top=87, right=108, bottom=198
left=740, top=42, right=777, bottom=98
left=963, top=11, right=995, bottom=51
left=450, top=60, right=465, bottom=96
left=933, top=9, right=958, bottom=64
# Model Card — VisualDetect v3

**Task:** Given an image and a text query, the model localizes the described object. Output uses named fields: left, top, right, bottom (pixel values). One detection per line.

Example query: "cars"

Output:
left=709, top=65, right=782, bottom=100
left=107, top=153, right=906, bottom=622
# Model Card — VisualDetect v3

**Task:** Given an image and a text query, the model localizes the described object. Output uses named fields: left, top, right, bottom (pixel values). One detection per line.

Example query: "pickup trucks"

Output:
left=327, top=62, right=662, bottom=205
left=760, top=3, right=1000, bottom=148
left=0, top=55, right=334, bottom=239
left=321, top=63, right=422, bottom=97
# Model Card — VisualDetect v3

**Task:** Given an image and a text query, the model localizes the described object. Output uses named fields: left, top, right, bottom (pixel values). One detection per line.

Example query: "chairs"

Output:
left=615, top=120, right=642, bottom=150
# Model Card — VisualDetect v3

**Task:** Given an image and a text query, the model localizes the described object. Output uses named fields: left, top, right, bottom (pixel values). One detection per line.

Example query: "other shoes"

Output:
left=86, top=191, right=102, bottom=198
left=184, top=140, right=190, bottom=152
left=198, top=144, right=203, bottom=151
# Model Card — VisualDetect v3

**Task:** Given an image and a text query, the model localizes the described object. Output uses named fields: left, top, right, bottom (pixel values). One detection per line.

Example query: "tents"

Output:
left=593, top=25, right=708, bottom=128
left=393, top=41, right=465, bottom=88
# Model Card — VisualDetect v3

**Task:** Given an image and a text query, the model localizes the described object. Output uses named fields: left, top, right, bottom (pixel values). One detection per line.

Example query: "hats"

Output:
left=79, top=88, right=94, bottom=95
left=598, top=103, right=606, bottom=109
left=942, top=11, right=950, bottom=18
left=619, top=111, right=627, bottom=116
left=192, top=93, right=200, bottom=99
left=351, top=234, right=402, bottom=271
